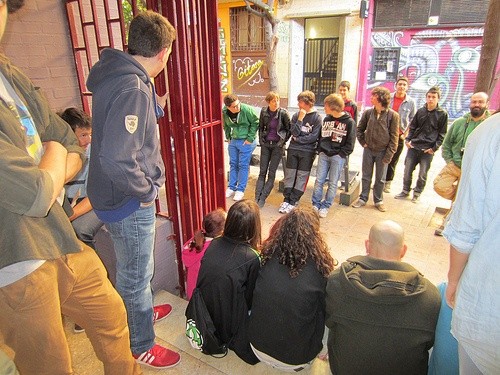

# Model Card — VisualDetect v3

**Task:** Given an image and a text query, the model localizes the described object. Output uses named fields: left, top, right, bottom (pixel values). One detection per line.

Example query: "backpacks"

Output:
left=184, top=288, right=226, bottom=355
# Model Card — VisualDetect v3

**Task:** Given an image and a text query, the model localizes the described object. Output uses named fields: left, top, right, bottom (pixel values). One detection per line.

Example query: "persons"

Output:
left=352, top=87, right=400, bottom=212
left=181, top=208, right=226, bottom=302
left=221, top=92, right=260, bottom=202
left=255, top=91, right=292, bottom=208
left=59, top=107, right=104, bottom=335
left=442, top=110, right=500, bottom=375
left=278, top=90, right=322, bottom=214
left=324, top=221, right=440, bottom=375
left=336, top=80, right=358, bottom=188
left=311, top=93, right=356, bottom=218
left=432, top=92, right=491, bottom=237
left=247, top=203, right=335, bottom=373
left=86, top=9, right=180, bottom=369
left=383, top=78, right=417, bottom=193
left=0, top=0, right=142, bottom=375
left=195, top=200, right=265, bottom=350
left=394, top=87, right=449, bottom=203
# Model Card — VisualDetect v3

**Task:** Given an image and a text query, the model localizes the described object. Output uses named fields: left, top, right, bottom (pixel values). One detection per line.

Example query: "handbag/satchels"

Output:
left=433, top=161, right=461, bottom=200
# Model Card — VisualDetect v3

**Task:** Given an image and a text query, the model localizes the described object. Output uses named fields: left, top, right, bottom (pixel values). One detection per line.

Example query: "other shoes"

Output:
left=75, top=323, right=85, bottom=333
left=384, top=185, right=391, bottom=193
left=395, top=192, right=408, bottom=199
left=413, top=195, right=418, bottom=202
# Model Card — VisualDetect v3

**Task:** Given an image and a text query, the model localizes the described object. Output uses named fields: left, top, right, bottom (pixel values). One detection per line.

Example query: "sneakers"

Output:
left=131, top=343, right=180, bottom=369
left=151, top=304, right=172, bottom=322
left=278, top=202, right=289, bottom=213
left=227, top=187, right=234, bottom=197
left=435, top=226, right=445, bottom=236
left=352, top=200, right=364, bottom=208
left=377, top=204, right=386, bottom=212
left=284, top=204, right=295, bottom=214
left=319, top=207, right=328, bottom=218
left=233, top=192, right=245, bottom=201
left=312, top=206, right=320, bottom=212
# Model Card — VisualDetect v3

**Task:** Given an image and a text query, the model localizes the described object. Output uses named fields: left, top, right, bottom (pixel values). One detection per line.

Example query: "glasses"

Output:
left=228, top=100, right=242, bottom=110
